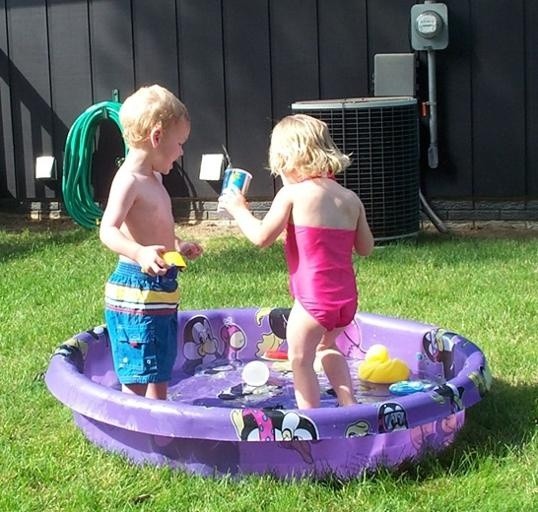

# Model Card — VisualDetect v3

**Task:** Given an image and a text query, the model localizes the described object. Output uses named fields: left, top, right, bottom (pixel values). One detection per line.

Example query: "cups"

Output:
left=215, top=168, right=252, bottom=225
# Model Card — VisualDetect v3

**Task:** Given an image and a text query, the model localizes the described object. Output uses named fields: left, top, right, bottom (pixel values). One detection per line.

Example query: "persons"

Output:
left=99, top=84, right=205, bottom=401
left=218, top=112, right=373, bottom=411
